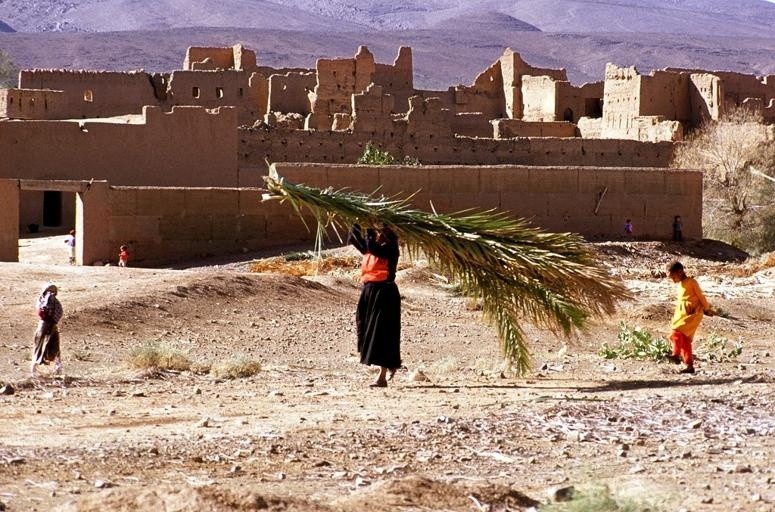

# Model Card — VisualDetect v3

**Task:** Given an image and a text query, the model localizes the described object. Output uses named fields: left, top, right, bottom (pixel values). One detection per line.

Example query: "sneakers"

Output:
left=369, top=377, right=388, bottom=387
left=680, top=365, right=695, bottom=374
left=664, top=353, right=681, bottom=366
left=388, top=368, right=397, bottom=381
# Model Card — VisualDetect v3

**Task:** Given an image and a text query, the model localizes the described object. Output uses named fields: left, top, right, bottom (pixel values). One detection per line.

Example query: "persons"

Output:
left=350, top=217, right=403, bottom=388
left=34, top=285, right=63, bottom=365
left=673, top=214, right=685, bottom=242
left=67, top=230, right=76, bottom=263
left=623, top=218, right=636, bottom=239
left=115, top=245, right=130, bottom=267
left=665, top=261, right=711, bottom=373
